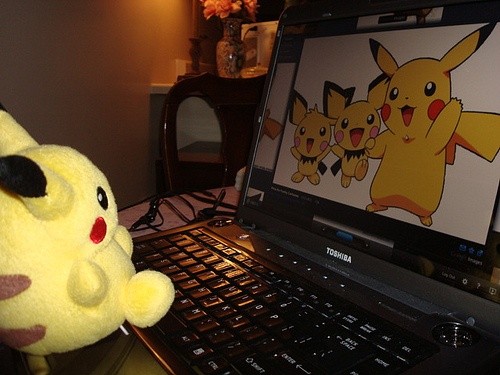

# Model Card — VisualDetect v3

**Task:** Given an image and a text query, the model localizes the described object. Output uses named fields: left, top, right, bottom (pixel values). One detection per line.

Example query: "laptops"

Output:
left=121, top=0, right=498, bottom=375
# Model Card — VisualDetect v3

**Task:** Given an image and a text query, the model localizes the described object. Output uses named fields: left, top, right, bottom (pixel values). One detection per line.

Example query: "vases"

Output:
left=216, top=17, right=244, bottom=79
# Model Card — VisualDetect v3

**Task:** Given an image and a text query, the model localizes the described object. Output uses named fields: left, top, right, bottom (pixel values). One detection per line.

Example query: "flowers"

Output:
left=201, top=0, right=261, bottom=25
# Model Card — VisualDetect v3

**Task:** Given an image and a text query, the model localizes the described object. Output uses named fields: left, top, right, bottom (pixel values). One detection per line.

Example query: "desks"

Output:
left=148, top=85, right=262, bottom=200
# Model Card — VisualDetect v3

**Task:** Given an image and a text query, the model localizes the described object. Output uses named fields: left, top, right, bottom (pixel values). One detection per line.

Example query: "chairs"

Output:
left=162, top=70, right=252, bottom=195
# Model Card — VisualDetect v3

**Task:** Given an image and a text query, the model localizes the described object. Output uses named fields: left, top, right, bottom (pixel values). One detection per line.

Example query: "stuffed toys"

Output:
left=0, top=104, right=174, bottom=370
